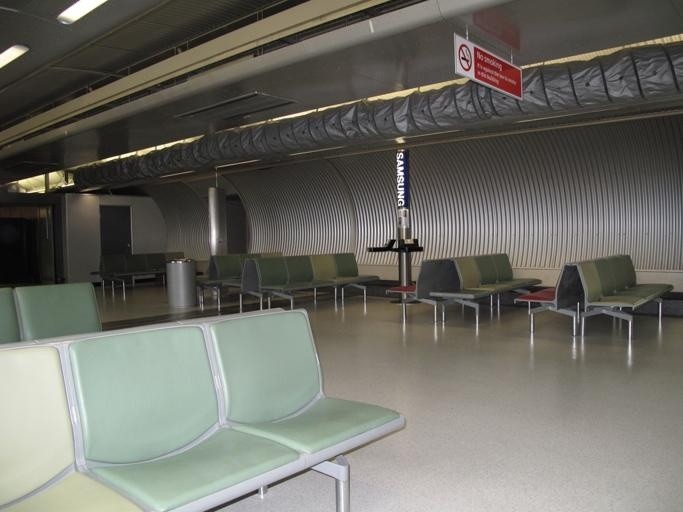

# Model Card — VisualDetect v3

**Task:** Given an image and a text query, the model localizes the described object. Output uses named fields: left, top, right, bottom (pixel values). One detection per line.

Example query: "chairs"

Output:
left=512, top=252, right=672, bottom=347
left=1, top=306, right=405, bottom=512
left=382, top=252, right=544, bottom=338
left=0, top=284, right=407, bottom=511
left=95, top=252, right=193, bottom=306
left=195, top=251, right=382, bottom=320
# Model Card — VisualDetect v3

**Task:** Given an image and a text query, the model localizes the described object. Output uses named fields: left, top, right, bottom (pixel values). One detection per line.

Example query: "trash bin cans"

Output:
left=165, top=258, right=198, bottom=309
